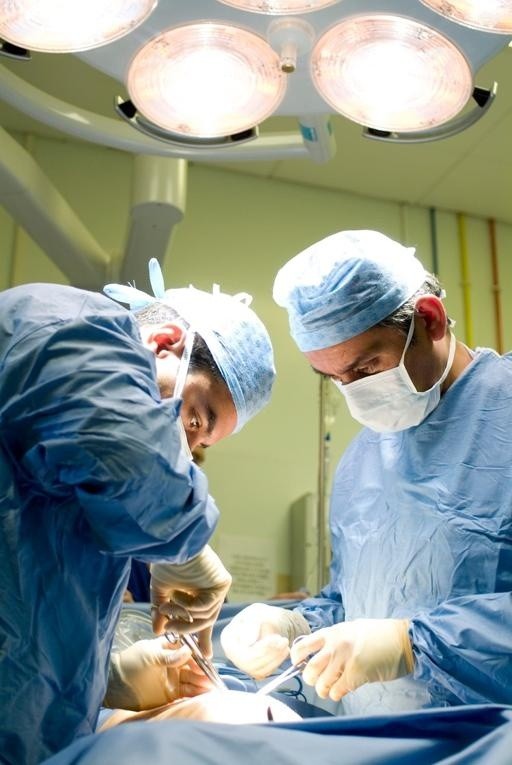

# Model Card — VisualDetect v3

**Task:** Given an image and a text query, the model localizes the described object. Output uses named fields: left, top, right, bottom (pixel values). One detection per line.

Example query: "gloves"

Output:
left=103, top=634, right=212, bottom=711
left=221, top=603, right=312, bottom=680
left=290, top=618, right=413, bottom=700
left=150, top=544, right=232, bottom=660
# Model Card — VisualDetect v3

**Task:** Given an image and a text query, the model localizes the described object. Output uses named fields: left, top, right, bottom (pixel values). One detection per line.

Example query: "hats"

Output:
left=160, top=287, right=276, bottom=436
left=272, top=230, right=426, bottom=352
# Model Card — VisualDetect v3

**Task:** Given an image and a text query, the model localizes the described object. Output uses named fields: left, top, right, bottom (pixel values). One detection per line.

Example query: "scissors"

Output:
left=151, top=599, right=229, bottom=691
left=256, top=635, right=321, bottom=696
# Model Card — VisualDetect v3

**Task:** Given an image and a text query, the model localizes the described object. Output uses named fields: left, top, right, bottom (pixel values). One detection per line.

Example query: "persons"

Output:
left=0, top=284, right=276, bottom=765
left=220, top=229, right=512, bottom=705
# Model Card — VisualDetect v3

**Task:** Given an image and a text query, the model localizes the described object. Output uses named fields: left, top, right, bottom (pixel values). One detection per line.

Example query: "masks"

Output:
left=331, top=366, right=440, bottom=433
